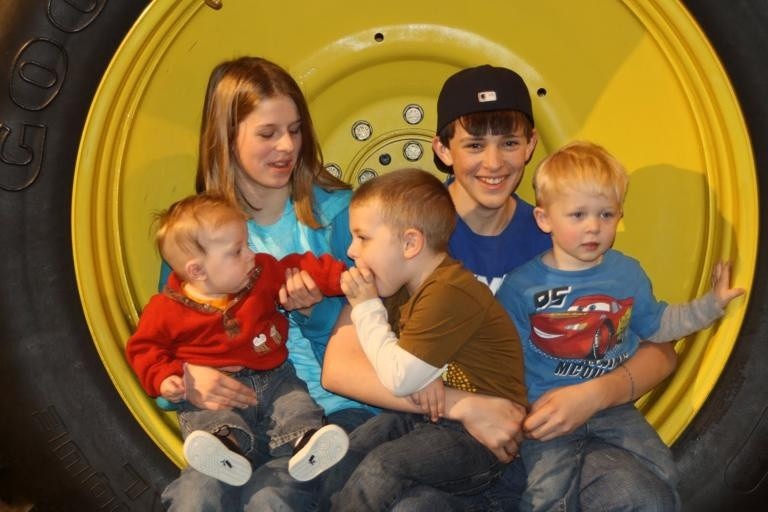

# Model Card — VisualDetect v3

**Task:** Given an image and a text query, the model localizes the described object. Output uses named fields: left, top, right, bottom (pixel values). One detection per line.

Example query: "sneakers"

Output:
left=288, top=425, right=350, bottom=482
left=183, top=431, right=252, bottom=489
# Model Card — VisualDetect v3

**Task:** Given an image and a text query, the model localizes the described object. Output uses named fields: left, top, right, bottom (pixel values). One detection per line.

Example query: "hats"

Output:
left=433, top=64, right=533, bottom=174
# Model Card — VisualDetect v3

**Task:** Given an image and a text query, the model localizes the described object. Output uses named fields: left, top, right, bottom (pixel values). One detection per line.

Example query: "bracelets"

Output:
left=619, top=363, right=634, bottom=403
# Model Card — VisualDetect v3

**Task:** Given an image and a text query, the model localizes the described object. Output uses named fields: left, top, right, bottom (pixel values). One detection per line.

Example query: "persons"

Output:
left=493, top=142, right=745, bottom=512
left=155, top=54, right=377, bottom=512
left=324, top=64, right=684, bottom=512
left=126, top=193, right=347, bottom=488
left=323, top=170, right=527, bottom=512
